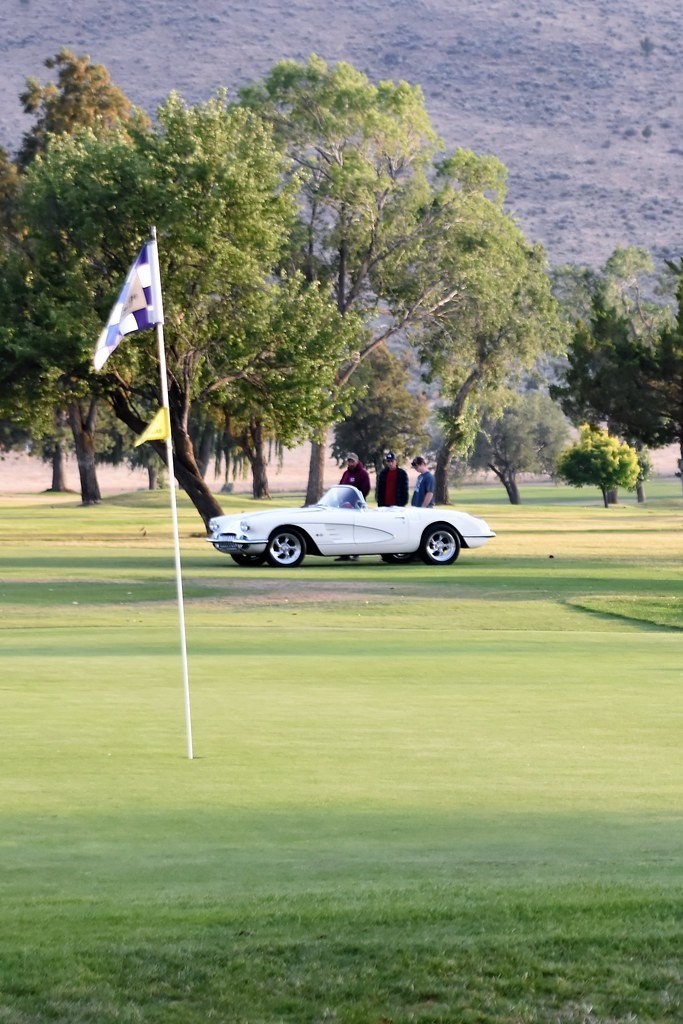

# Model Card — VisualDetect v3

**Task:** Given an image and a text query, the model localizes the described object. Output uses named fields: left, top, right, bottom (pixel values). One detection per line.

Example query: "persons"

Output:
left=335, top=453, right=371, bottom=562
left=375, top=451, right=409, bottom=508
left=411, top=456, right=435, bottom=508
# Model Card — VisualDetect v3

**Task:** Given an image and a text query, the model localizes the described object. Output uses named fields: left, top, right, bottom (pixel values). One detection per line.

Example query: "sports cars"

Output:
left=206, top=481, right=498, bottom=569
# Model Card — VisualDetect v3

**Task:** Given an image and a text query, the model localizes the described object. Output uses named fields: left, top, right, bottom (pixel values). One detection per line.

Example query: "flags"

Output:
left=93, top=239, right=157, bottom=372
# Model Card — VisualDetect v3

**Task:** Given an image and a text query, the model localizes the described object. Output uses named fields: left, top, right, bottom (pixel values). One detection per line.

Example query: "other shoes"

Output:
left=335, top=557, right=350, bottom=561
left=351, top=556, right=360, bottom=561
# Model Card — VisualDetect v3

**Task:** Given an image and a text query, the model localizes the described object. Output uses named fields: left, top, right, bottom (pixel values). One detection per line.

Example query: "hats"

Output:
left=385, top=452, right=395, bottom=461
left=347, top=452, right=359, bottom=464
